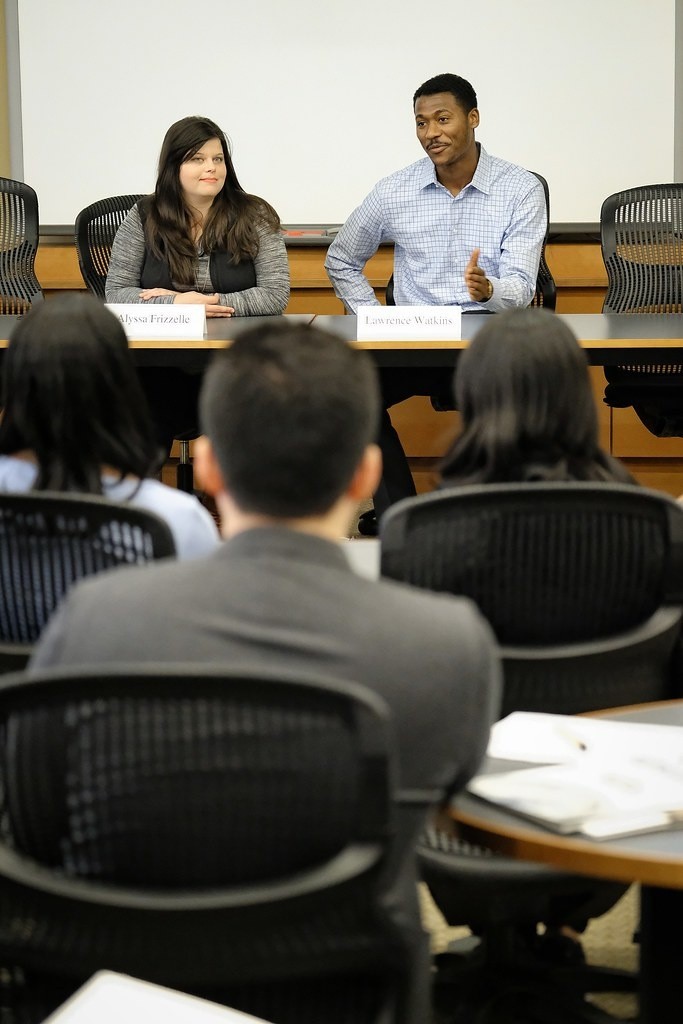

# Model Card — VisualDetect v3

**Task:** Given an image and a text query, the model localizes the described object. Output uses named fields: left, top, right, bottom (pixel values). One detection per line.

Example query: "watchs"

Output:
left=487, top=281, right=492, bottom=294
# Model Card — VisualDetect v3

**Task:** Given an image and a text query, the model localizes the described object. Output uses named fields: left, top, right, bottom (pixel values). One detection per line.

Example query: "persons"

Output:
left=427, top=309, right=643, bottom=972
left=323, top=73, right=548, bottom=538
left=0, top=290, right=222, bottom=565
left=26, top=321, right=503, bottom=1024
left=104, top=116, right=291, bottom=319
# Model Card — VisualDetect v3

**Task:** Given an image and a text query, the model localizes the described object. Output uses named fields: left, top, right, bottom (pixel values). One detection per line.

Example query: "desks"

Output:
left=438, top=701, right=683, bottom=888
left=1, top=314, right=683, bottom=347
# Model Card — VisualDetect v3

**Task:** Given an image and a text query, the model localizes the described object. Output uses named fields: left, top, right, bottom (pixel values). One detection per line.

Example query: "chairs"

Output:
left=75, top=193, right=203, bottom=492
left=384, top=170, right=558, bottom=411
left=0, top=177, right=45, bottom=315
left=601, top=183, right=683, bottom=454
left=0, top=664, right=395, bottom=1024
left=0, top=493, right=176, bottom=681
left=378, top=478, right=683, bottom=716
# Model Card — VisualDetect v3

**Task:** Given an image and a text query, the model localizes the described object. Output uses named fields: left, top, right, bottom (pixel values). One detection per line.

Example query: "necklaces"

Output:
left=194, top=248, right=212, bottom=292
